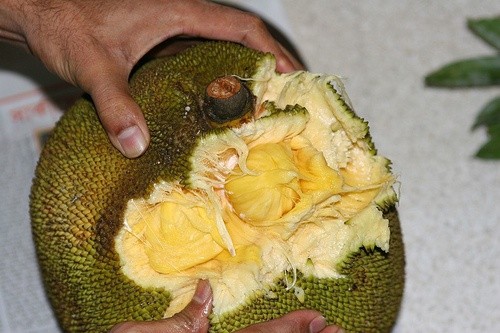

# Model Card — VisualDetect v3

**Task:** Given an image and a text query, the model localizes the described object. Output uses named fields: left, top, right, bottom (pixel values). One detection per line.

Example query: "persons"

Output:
left=0, top=0, right=347, bottom=333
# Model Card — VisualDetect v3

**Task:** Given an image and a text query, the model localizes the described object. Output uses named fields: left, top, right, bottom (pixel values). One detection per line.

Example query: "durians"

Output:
left=30, top=40, right=407, bottom=333
